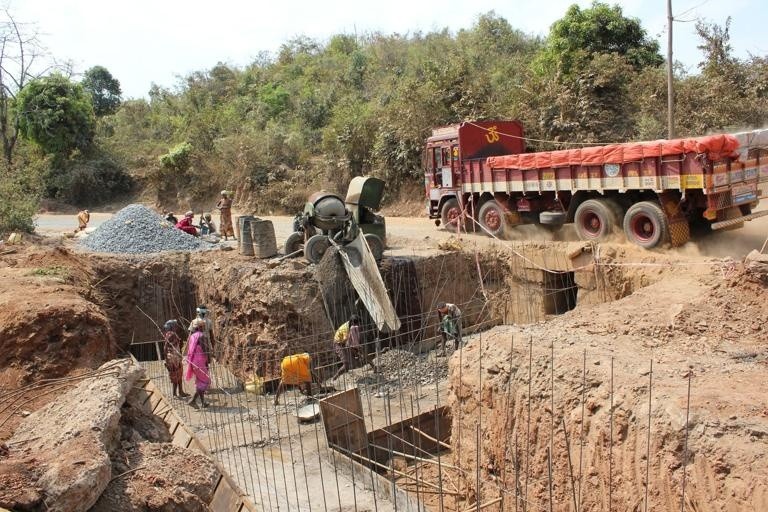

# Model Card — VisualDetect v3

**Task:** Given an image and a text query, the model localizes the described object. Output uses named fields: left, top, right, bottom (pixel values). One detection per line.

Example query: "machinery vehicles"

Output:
left=284, top=176, right=386, bottom=267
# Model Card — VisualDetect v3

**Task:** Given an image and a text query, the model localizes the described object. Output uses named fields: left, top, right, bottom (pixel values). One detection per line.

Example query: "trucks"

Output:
left=424, top=118, right=768, bottom=250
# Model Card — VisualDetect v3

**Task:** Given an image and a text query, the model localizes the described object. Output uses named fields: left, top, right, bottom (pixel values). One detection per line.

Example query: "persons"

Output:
left=332, top=313, right=360, bottom=381
left=165, top=212, right=178, bottom=225
left=177, top=210, right=200, bottom=236
left=186, top=319, right=210, bottom=409
left=188, top=306, right=215, bottom=372
left=163, top=318, right=191, bottom=397
left=78, top=209, right=89, bottom=231
left=199, top=213, right=216, bottom=234
left=274, top=352, right=322, bottom=406
left=216, top=190, right=237, bottom=241
left=437, top=302, right=462, bottom=357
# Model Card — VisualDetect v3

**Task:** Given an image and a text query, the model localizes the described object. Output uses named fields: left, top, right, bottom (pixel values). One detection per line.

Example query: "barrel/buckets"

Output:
left=250, top=219, right=278, bottom=259
left=235, top=215, right=258, bottom=255
